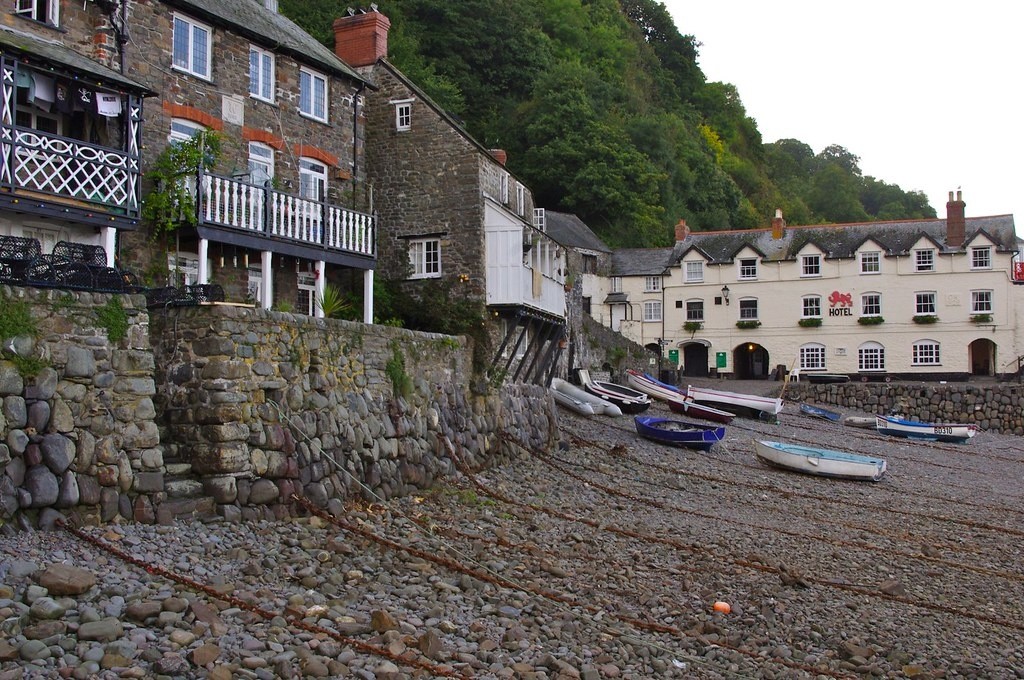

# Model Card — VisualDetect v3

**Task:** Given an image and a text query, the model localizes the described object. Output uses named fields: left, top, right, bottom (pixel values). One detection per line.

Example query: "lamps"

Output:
left=721, top=285, right=730, bottom=305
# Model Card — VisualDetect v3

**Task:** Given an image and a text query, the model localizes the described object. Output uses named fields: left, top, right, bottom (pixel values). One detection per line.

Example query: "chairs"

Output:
left=789, top=368, right=800, bottom=383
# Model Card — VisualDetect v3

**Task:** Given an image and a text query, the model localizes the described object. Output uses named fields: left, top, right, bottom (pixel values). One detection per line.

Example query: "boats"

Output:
left=843, top=413, right=905, bottom=431
left=584, top=381, right=647, bottom=415
left=688, top=356, right=796, bottom=424
left=667, top=397, right=737, bottom=425
left=753, top=437, right=888, bottom=482
left=547, top=377, right=623, bottom=418
left=633, top=416, right=726, bottom=450
left=875, top=413, right=979, bottom=444
left=644, top=373, right=689, bottom=396
left=592, top=379, right=648, bottom=403
left=626, top=368, right=694, bottom=405
left=800, top=402, right=842, bottom=422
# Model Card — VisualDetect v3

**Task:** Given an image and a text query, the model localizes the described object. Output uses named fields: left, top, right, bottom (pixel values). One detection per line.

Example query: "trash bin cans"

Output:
left=710, top=367, right=718, bottom=379
left=662, top=369, right=675, bottom=386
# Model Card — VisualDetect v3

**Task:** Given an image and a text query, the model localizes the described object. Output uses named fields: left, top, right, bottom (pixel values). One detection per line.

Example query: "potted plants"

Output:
left=564, top=266, right=576, bottom=293
